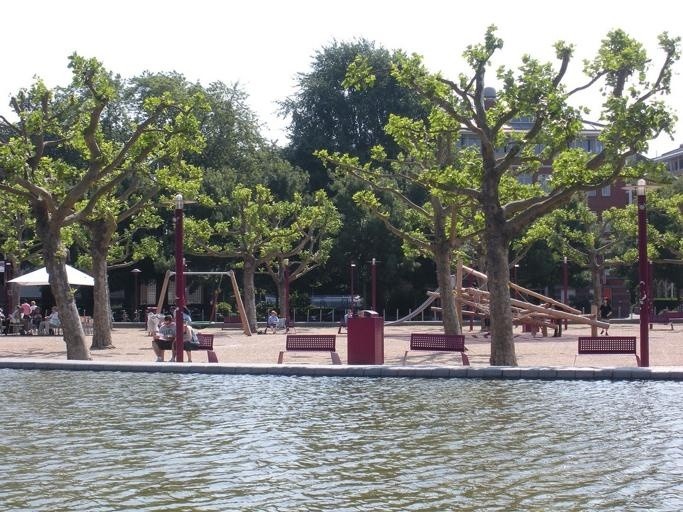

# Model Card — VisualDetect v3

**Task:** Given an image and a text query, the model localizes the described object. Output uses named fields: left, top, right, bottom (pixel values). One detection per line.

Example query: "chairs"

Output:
left=0, top=312, right=94, bottom=336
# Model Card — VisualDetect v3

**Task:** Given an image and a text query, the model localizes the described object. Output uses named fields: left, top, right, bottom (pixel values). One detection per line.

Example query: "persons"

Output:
left=599, top=297, right=612, bottom=335
left=345, top=309, right=353, bottom=330
left=0, top=299, right=61, bottom=336
left=268, top=310, right=279, bottom=334
left=552, top=297, right=563, bottom=337
left=147, top=302, right=200, bottom=362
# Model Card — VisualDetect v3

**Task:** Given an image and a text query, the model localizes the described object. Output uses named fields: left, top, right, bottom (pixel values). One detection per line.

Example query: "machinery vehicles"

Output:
left=259, top=292, right=363, bottom=319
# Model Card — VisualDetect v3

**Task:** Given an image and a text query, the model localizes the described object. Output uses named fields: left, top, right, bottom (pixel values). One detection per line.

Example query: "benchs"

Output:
left=157, top=334, right=217, bottom=363
left=220, top=316, right=242, bottom=330
left=647, top=312, right=673, bottom=330
left=275, top=334, right=340, bottom=366
left=335, top=319, right=347, bottom=333
left=264, top=315, right=289, bottom=333
left=571, top=336, right=640, bottom=366
left=399, top=332, right=469, bottom=364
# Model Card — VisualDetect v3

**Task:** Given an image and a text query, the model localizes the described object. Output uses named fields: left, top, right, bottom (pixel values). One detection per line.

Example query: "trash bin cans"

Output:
left=346, top=310, right=383, bottom=365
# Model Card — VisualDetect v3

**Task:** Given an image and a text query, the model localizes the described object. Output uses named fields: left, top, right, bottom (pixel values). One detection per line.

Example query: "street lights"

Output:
left=563, top=255, right=569, bottom=304
left=513, top=263, right=521, bottom=302
left=347, top=259, right=356, bottom=318
left=370, top=257, right=379, bottom=312
left=129, top=267, right=142, bottom=322
left=172, top=193, right=187, bottom=361
left=633, top=180, right=657, bottom=367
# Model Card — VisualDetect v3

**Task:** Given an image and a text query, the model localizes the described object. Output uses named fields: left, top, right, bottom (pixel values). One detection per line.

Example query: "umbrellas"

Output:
left=8, top=263, right=95, bottom=287
